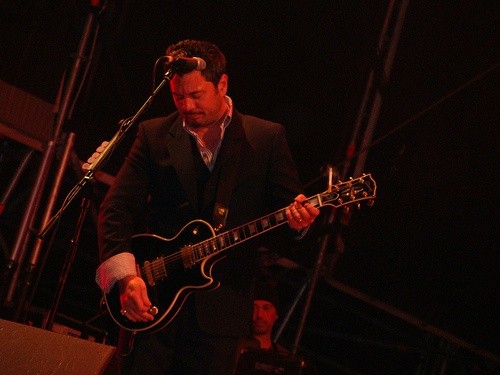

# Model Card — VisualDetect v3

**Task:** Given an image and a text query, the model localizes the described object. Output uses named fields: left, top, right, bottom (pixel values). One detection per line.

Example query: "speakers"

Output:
left=0, top=319, right=124, bottom=375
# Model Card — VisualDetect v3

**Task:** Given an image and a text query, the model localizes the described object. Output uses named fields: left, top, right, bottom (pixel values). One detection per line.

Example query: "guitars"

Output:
left=103, top=170, right=376, bottom=332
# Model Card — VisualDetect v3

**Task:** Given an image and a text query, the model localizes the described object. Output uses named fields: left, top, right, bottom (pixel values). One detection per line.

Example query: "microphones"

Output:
left=161, top=55, right=206, bottom=70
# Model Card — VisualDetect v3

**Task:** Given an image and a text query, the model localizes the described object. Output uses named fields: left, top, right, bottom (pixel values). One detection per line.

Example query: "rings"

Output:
left=123, top=309, right=128, bottom=316
left=298, top=219, right=303, bottom=222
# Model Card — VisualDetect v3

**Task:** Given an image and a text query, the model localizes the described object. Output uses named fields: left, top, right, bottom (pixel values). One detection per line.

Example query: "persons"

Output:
left=230, top=282, right=307, bottom=375
left=96, top=40, right=321, bottom=375
